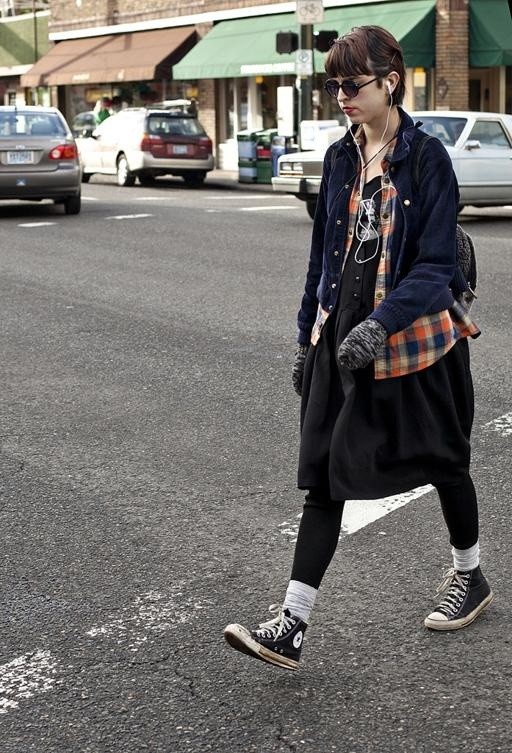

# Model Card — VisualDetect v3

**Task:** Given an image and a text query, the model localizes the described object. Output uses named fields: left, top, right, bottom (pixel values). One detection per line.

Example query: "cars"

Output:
left=0, top=106, right=83, bottom=214
left=270, top=111, right=512, bottom=219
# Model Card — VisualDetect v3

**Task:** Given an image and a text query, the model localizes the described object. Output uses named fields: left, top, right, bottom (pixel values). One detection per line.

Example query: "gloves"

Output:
left=292, top=343, right=309, bottom=395
left=338, top=319, right=387, bottom=370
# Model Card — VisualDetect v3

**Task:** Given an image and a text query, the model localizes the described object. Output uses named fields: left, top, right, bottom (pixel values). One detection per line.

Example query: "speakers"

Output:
left=314, top=30, right=338, bottom=53
left=276, top=32, right=298, bottom=54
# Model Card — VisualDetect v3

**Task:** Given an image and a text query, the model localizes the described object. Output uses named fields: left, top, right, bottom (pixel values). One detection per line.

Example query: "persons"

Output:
left=220, top=21, right=497, bottom=672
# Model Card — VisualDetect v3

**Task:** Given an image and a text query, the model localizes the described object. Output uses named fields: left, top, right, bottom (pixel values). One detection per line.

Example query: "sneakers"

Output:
left=224, top=608, right=307, bottom=670
left=425, top=565, right=492, bottom=631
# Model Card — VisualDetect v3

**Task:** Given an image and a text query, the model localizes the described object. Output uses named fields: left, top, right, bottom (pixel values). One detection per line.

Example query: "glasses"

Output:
left=324, top=76, right=383, bottom=98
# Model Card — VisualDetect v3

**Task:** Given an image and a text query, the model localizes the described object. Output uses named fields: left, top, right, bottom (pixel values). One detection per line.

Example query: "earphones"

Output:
left=386, top=80, right=391, bottom=95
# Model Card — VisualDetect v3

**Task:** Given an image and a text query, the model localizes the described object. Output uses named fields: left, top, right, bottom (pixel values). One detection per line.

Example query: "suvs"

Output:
left=75, top=106, right=214, bottom=187
